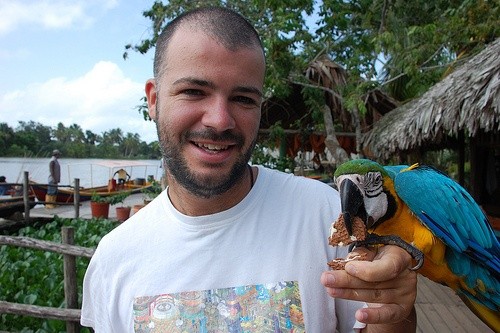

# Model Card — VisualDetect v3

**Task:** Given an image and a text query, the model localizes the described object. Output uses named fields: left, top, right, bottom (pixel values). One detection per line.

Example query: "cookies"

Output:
left=327, top=213, right=366, bottom=246
left=327, top=248, right=368, bottom=271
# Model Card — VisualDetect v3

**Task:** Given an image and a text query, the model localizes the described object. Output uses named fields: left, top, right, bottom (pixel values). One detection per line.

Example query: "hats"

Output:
left=52, top=149, right=62, bottom=154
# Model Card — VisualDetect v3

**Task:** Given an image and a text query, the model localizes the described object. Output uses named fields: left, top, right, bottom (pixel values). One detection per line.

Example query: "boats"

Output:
left=27, top=179, right=152, bottom=203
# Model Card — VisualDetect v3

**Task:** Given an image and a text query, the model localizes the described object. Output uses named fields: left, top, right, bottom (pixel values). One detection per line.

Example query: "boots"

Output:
left=45, top=195, right=57, bottom=208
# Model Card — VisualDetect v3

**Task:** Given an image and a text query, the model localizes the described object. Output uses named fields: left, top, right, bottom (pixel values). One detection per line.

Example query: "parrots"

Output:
left=333, top=159, right=500, bottom=314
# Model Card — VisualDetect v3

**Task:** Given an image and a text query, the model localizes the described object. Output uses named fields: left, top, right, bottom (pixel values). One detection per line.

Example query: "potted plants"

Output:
left=89, top=175, right=165, bottom=220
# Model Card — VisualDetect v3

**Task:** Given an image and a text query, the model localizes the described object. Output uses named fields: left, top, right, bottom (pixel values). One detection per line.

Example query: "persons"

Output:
left=45, top=150, right=62, bottom=208
left=78, top=7, right=418, bottom=333
left=112, top=168, right=132, bottom=191
left=0, top=176, right=19, bottom=196
